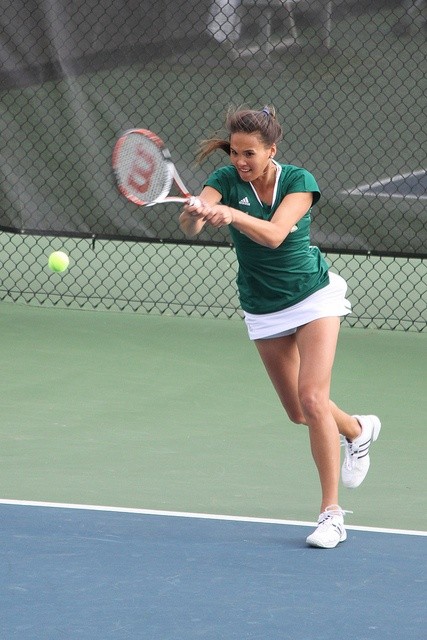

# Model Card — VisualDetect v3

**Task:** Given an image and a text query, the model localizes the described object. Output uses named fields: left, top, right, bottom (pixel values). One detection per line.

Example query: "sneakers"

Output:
left=306, top=504, right=353, bottom=548
left=340, top=415, right=381, bottom=488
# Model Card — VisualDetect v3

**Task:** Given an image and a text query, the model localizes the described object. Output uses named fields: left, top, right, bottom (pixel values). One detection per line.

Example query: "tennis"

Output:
left=48, top=251, right=70, bottom=273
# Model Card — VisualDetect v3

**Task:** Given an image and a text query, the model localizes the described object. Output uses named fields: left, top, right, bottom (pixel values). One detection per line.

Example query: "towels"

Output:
left=207, top=1, right=240, bottom=43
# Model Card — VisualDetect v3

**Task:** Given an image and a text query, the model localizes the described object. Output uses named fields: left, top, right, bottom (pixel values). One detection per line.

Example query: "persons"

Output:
left=178, top=105, right=380, bottom=550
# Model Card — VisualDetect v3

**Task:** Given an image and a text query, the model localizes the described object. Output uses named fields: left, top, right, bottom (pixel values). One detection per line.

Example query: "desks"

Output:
left=227, top=1, right=332, bottom=56
